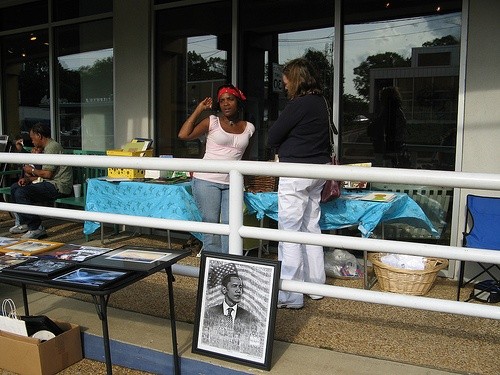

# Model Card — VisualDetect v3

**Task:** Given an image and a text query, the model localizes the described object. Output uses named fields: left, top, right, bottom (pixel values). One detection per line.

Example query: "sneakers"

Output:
left=9, top=224, right=27, bottom=233
left=22, top=225, right=46, bottom=239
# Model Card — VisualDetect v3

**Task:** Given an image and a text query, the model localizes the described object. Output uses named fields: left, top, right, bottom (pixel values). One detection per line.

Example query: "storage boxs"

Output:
left=106, top=149, right=146, bottom=180
left=0, top=319, right=83, bottom=375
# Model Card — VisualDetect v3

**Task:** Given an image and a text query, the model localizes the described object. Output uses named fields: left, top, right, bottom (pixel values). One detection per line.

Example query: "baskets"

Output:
left=367, top=253, right=449, bottom=295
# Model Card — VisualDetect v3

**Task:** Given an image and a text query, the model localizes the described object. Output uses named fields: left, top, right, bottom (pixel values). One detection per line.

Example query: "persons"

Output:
left=268, top=58, right=331, bottom=307
left=367, top=87, right=407, bottom=167
left=178, top=84, right=255, bottom=253
left=9, top=122, right=74, bottom=239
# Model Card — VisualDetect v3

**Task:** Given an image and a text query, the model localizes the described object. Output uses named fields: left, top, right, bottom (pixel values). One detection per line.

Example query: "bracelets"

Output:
left=32, top=168, right=35, bottom=176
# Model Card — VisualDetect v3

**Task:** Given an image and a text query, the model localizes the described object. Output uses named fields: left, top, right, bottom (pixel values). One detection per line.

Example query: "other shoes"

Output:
left=277, top=303, right=303, bottom=309
left=306, top=293, right=324, bottom=300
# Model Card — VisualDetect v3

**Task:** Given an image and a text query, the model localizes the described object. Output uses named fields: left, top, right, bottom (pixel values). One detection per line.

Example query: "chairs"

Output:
left=457, top=193, right=500, bottom=303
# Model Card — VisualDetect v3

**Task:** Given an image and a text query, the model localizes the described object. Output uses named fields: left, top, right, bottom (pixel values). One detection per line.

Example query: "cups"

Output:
left=72, top=184, right=82, bottom=198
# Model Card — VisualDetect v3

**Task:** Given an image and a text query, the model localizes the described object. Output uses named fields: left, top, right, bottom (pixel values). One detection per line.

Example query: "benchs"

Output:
left=0, top=146, right=119, bottom=241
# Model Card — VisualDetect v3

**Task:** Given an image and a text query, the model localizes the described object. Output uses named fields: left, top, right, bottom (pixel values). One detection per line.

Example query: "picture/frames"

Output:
left=191, top=250, right=282, bottom=371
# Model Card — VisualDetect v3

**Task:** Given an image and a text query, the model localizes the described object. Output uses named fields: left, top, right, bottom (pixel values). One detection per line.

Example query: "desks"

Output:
left=243, top=189, right=434, bottom=291
left=0, top=238, right=190, bottom=375
left=83, top=175, right=200, bottom=250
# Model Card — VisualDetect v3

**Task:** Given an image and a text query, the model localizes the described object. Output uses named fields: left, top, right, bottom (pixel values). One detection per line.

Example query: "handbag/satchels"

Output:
left=322, top=153, right=341, bottom=203
left=0, top=298, right=28, bottom=337
